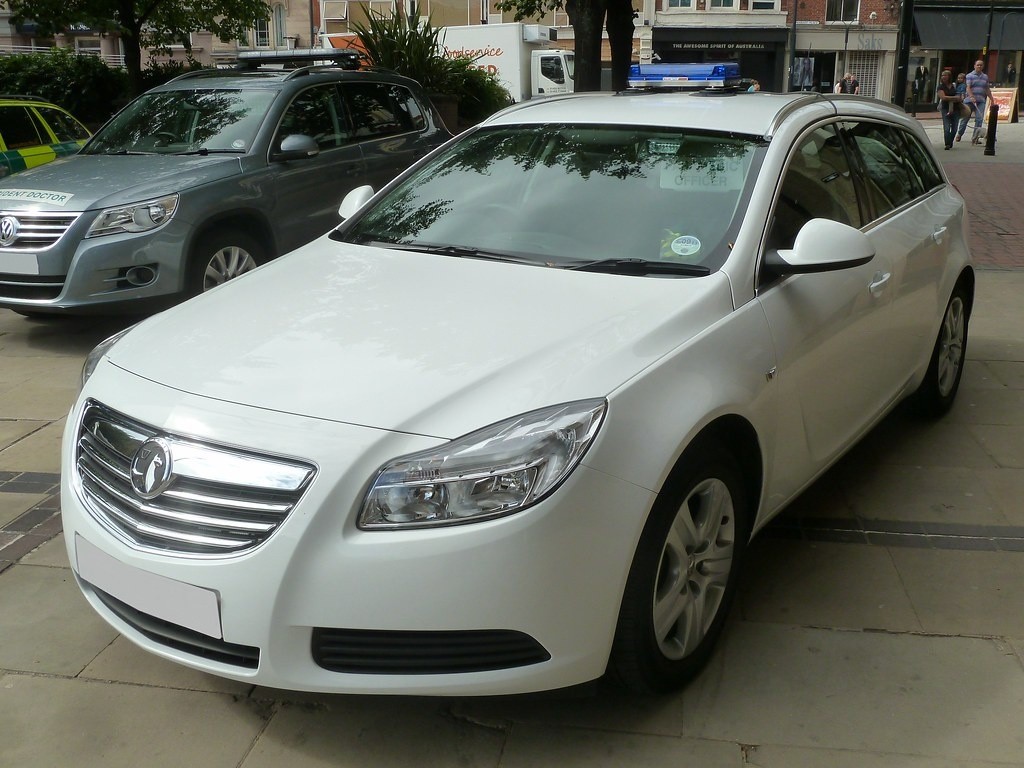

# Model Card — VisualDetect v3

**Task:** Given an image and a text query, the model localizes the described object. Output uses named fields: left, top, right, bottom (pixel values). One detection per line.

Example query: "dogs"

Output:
left=966, top=125, right=997, bottom=145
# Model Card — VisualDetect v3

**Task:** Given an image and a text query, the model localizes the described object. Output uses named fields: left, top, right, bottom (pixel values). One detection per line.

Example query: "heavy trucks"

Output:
left=317, top=21, right=576, bottom=104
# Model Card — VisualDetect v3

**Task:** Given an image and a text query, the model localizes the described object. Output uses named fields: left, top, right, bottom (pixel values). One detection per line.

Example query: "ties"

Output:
left=922, top=67, right=924, bottom=74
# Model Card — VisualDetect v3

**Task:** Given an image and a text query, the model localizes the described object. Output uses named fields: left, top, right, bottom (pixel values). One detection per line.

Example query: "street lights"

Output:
left=828, top=19, right=867, bottom=80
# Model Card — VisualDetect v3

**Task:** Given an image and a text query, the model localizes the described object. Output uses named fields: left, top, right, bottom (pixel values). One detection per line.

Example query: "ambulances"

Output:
left=1, top=92, right=95, bottom=179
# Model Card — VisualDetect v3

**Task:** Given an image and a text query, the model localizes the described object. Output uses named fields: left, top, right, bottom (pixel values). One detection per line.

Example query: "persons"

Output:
left=851, top=76, right=859, bottom=96
left=937, top=60, right=994, bottom=151
left=799, top=59, right=813, bottom=85
left=835, top=73, right=852, bottom=93
left=748, top=80, right=760, bottom=92
left=810, top=79, right=823, bottom=92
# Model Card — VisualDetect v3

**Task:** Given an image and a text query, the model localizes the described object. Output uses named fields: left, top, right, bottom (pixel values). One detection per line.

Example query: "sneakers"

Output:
left=974, top=139, right=982, bottom=144
left=956, top=135, right=962, bottom=142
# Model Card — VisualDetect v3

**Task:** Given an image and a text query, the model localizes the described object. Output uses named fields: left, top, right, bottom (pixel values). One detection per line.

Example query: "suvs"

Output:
left=0, top=48, right=457, bottom=317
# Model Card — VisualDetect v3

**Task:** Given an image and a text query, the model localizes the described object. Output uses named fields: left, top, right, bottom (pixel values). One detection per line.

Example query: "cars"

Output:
left=56, top=65, right=975, bottom=710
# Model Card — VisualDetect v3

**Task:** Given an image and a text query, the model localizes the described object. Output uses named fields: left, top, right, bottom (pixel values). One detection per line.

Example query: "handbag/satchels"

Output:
left=959, top=101, right=971, bottom=119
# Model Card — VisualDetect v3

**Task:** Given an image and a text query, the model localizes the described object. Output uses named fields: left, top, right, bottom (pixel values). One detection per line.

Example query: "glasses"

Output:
left=1008, top=66, right=1012, bottom=69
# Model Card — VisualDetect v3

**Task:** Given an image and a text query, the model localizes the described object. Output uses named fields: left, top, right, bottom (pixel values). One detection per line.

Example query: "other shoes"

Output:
left=944, top=145, right=952, bottom=150
